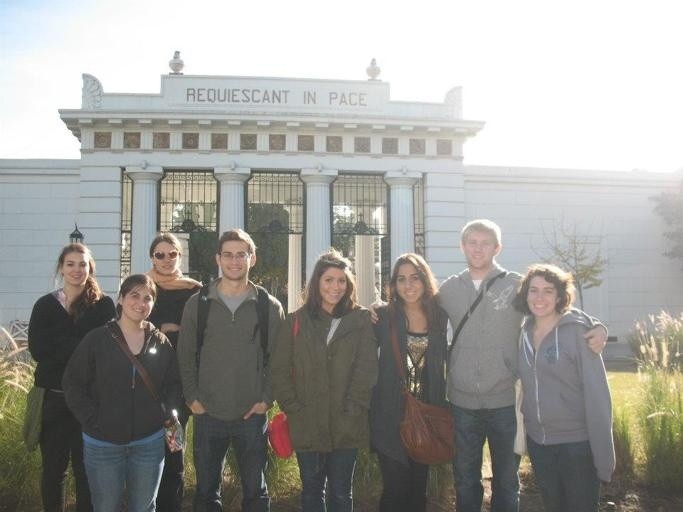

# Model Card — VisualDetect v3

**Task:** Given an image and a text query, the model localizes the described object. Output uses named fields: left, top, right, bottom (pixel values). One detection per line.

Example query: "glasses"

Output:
left=152, top=251, right=178, bottom=260
left=220, top=250, right=250, bottom=262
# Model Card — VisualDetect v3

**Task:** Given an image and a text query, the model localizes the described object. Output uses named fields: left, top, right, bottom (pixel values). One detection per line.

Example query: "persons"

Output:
left=60, top=274, right=183, bottom=512
left=143, top=232, right=203, bottom=512
left=370, top=252, right=449, bottom=512
left=27, top=243, right=116, bottom=512
left=176, top=229, right=285, bottom=512
left=511, top=263, right=616, bottom=512
left=369, top=219, right=609, bottom=512
left=271, top=247, right=380, bottom=512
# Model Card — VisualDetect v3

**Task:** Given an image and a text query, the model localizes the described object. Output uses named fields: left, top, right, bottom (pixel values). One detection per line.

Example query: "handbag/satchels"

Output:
left=164, top=409, right=184, bottom=454
left=513, top=412, right=527, bottom=455
left=400, top=394, right=454, bottom=465
left=269, top=413, right=293, bottom=459
